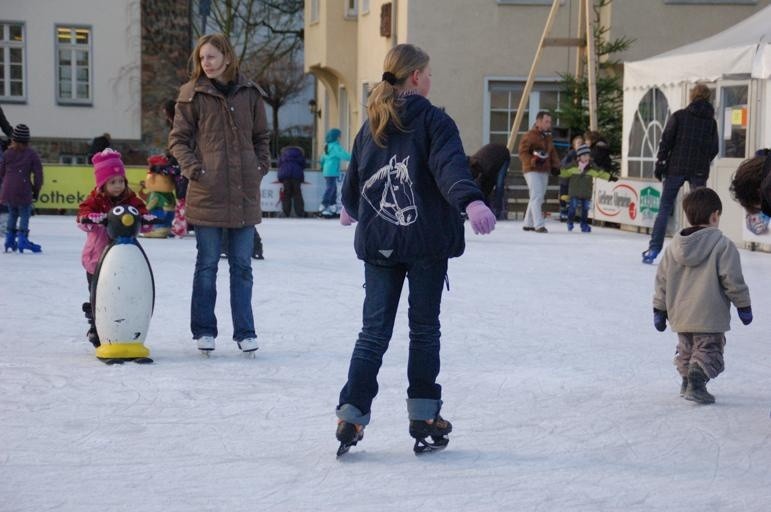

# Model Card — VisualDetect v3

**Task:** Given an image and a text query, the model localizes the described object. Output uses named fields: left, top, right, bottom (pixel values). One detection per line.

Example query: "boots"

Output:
left=5, top=229, right=17, bottom=252
left=679, top=363, right=715, bottom=403
left=18, top=231, right=41, bottom=252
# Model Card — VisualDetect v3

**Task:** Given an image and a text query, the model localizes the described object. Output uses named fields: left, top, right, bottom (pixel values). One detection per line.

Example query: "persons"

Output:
left=642, top=83, right=721, bottom=267
left=72, top=149, right=155, bottom=346
left=516, top=110, right=616, bottom=236
left=166, top=32, right=271, bottom=350
left=733, top=148, right=771, bottom=234
left=164, top=100, right=177, bottom=130
left=335, top=47, right=498, bottom=440
left=466, top=142, right=510, bottom=222
left=319, top=128, right=354, bottom=214
left=650, top=186, right=754, bottom=403
left=0, top=122, right=45, bottom=253
left=1, top=110, right=16, bottom=136
left=276, top=140, right=308, bottom=218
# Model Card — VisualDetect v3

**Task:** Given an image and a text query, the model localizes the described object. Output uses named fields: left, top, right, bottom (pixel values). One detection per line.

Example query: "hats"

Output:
left=326, top=129, right=341, bottom=143
left=92, top=149, right=128, bottom=191
left=576, top=145, right=591, bottom=156
left=12, top=123, right=31, bottom=144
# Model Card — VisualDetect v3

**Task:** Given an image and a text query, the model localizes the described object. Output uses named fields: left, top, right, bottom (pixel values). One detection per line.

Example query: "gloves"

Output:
left=738, top=306, right=752, bottom=325
left=654, top=310, right=669, bottom=331
left=609, top=176, right=618, bottom=181
left=655, top=161, right=666, bottom=180
left=340, top=206, right=356, bottom=226
left=536, top=158, right=544, bottom=166
left=551, top=168, right=561, bottom=175
left=466, top=201, right=496, bottom=236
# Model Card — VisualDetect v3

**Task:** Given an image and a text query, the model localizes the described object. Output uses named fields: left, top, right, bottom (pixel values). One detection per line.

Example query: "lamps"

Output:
left=306, top=97, right=322, bottom=118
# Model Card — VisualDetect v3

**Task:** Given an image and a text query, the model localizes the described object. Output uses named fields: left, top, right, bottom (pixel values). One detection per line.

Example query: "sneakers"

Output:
left=409, top=416, right=452, bottom=437
left=523, top=226, right=534, bottom=230
left=239, top=338, right=259, bottom=351
left=580, top=224, right=591, bottom=231
left=536, top=226, right=547, bottom=232
left=337, top=421, right=365, bottom=441
left=566, top=222, right=573, bottom=229
left=197, top=336, right=215, bottom=350
left=642, top=248, right=658, bottom=259
left=88, top=323, right=100, bottom=344
